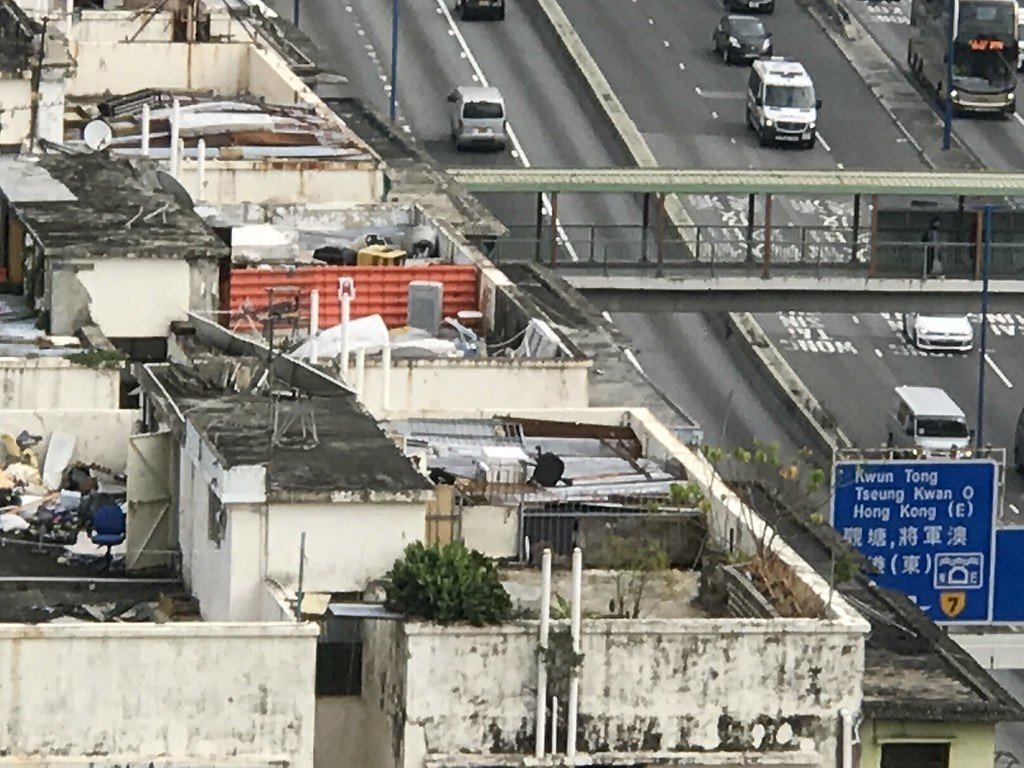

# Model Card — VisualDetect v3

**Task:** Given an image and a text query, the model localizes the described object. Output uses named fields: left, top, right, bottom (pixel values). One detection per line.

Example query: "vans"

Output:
left=448, top=85, right=508, bottom=153
left=885, top=383, right=975, bottom=461
left=746, top=55, right=822, bottom=149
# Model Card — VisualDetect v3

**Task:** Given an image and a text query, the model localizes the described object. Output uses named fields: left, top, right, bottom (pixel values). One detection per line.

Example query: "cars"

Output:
left=1014, top=407, right=1024, bottom=473
left=713, top=15, right=773, bottom=66
left=902, top=311, right=974, bottom=353
left=455, top=0, right=505, bottom=21
left=723, top=0, right=775, bottom=15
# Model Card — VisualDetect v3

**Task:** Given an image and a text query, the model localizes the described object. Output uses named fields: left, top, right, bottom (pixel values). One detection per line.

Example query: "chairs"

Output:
left=86, top=504, right=126, bottom=567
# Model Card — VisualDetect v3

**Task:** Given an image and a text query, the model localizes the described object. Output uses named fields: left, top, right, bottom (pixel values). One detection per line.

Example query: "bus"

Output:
left=907, top=0, right=1024, bottom=116
